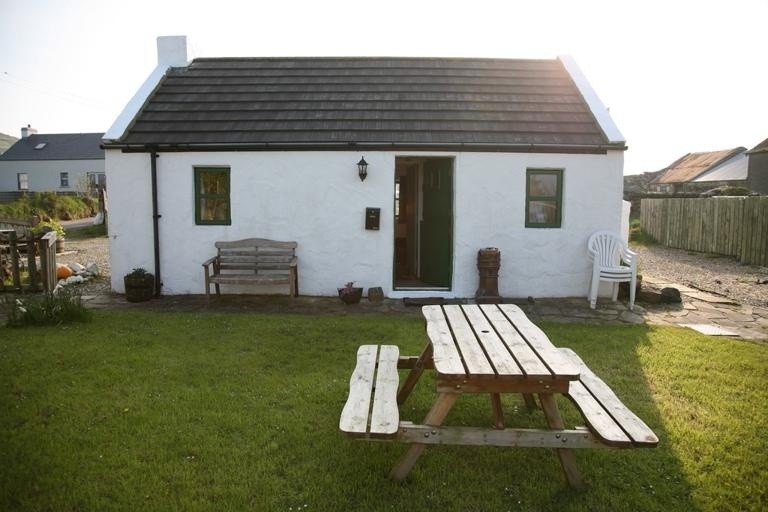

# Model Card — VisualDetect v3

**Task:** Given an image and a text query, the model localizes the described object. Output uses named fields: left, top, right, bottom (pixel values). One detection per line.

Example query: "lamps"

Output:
left=357, top=155, right=367, bottom=182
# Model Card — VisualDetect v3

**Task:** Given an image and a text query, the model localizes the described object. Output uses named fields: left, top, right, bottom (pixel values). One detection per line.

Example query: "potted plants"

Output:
left=124, top=267, right=154, bottom=303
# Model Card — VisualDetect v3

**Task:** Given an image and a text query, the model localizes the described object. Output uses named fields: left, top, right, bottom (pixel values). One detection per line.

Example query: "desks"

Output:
left=391, top=304, right=585, bottom=490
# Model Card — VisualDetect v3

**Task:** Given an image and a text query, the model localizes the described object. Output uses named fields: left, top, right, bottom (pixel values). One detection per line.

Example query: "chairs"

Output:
left=587, top=230, right=638, bottom=309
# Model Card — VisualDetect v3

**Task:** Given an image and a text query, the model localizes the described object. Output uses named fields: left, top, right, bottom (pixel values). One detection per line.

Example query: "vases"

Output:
left=337, top=288, right=362, bottom=304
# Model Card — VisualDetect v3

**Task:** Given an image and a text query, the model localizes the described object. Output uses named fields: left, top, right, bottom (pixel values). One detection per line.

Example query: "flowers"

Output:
left=341, top=280, right=352, bottom=297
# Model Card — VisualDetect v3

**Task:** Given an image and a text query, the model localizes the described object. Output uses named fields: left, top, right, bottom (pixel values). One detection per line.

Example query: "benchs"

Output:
left=202, top=237, right=297, bottom=307
left=337, top=343, right=415, bottom=442
left=556, top=347, right=659, bottom=449
